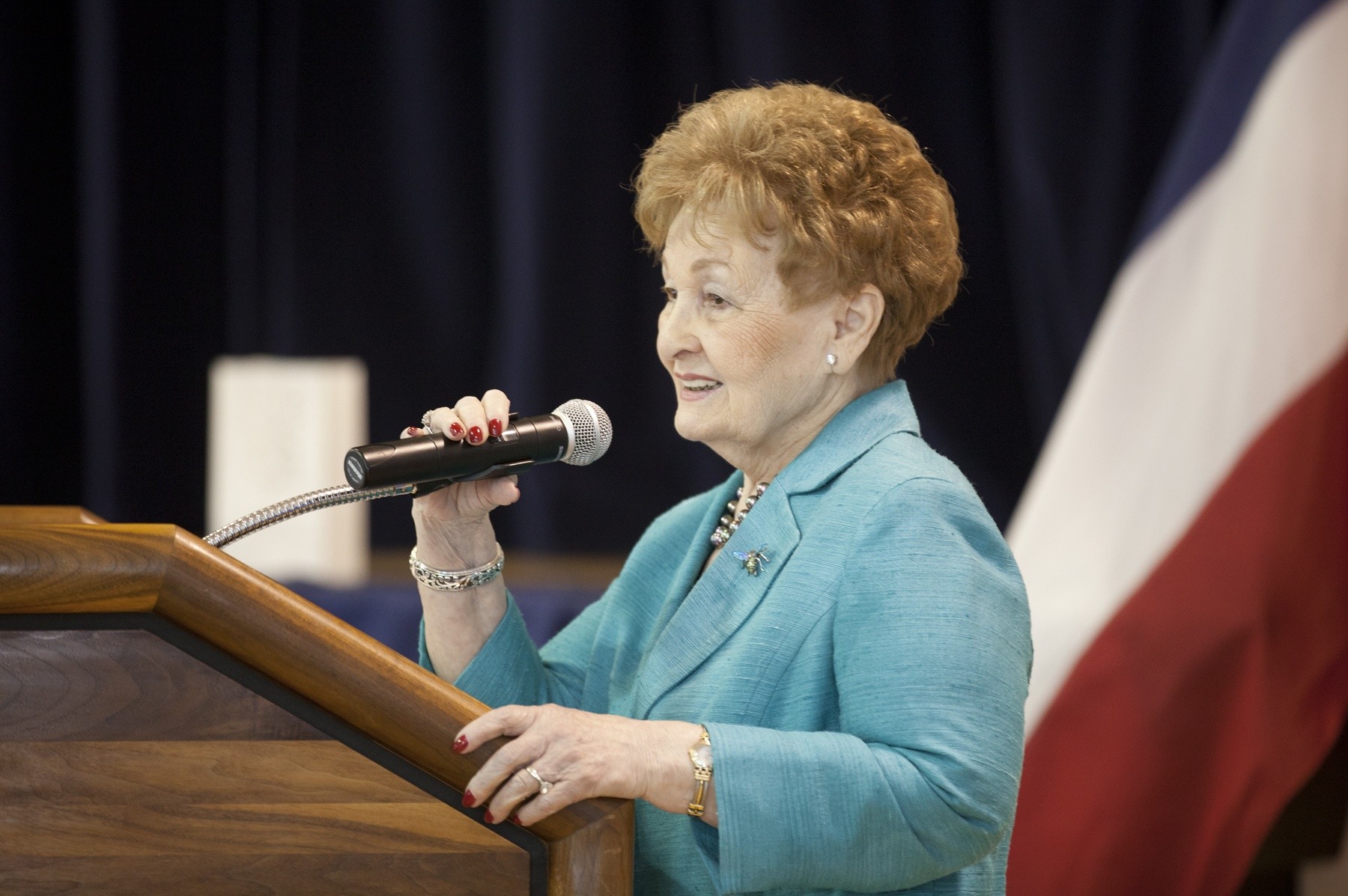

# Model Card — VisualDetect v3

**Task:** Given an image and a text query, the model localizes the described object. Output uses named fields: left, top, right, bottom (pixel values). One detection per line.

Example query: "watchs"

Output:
left=687, top=728, right=714, bottom=814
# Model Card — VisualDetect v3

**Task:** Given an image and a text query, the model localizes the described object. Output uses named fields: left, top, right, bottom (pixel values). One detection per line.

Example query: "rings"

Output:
left=525, top=765, right=553, bottom=795
left=421, top=411, right=438, bottom=434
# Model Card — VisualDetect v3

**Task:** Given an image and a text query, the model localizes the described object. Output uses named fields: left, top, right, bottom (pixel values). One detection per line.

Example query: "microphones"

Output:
left=343, top=398, right=614, bottom=491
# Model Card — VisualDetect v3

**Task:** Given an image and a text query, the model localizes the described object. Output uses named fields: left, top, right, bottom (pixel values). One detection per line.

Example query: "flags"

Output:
left=1001, top=4, right=1348, bottom=892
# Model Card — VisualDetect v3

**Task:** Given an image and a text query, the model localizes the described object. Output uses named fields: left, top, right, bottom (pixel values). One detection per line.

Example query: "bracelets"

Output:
left=408, top=541, right=506, bottom=591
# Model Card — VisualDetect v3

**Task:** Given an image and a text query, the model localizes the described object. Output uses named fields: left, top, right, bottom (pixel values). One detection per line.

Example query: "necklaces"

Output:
left=710, top=481, right=770, bottom=545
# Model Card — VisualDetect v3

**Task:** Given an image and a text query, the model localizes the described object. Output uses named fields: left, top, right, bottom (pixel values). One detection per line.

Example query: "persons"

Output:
left=400, top=82, right=1032, bottom=895
